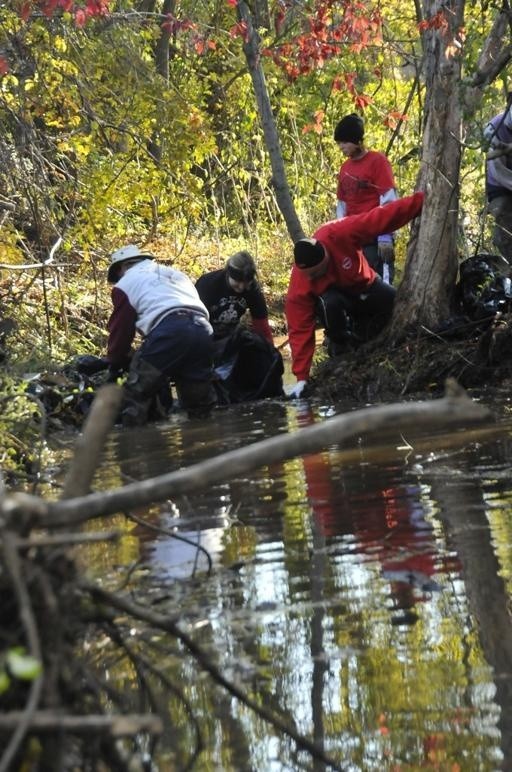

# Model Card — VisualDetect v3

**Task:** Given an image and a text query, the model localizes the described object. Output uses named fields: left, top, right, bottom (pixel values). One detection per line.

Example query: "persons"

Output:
left=105, top=245, right=214, bottom=426
left=284, top=192, right=424, bottom=400
left=482, top=112, right=511, bottom=265
left=195, top=248, right=274, bottom=347
left=334, top=114, right=397, bottom=284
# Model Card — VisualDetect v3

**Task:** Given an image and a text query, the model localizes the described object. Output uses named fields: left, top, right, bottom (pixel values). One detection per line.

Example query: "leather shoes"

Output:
left=377, top=239, right=397, bottom=266
left=286, top=380, right=311, bottom=399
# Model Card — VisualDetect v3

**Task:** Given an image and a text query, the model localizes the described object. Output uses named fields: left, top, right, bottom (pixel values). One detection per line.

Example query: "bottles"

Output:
left=293, top=237, right=328, bottom=270
left=227, top=249, right=256, bottom=284
left=333, top=112, right=366, bottom=145
left=106, top=246, right=156, bottom=284
